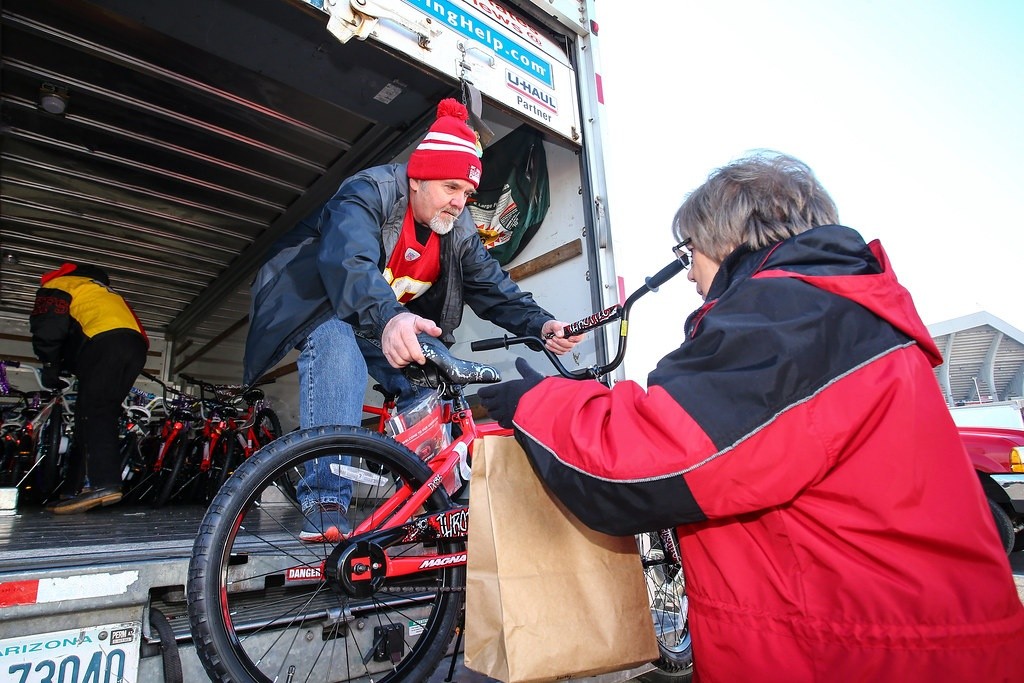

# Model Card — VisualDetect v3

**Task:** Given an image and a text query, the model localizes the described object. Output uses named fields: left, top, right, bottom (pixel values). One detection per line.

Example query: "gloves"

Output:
left=40, top=361, right=74, bottom=389
left=477, top=356, right=544, bottom=430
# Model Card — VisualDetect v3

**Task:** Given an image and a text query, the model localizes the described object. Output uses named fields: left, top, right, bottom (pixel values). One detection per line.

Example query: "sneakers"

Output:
left=298, top=502, right=353, bottom=542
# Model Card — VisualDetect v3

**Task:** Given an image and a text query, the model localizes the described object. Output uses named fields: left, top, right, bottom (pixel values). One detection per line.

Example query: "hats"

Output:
left=407, top=97, right=484, bottom=189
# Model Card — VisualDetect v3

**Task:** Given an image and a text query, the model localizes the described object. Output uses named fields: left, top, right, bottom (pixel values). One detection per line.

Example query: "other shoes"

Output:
left=55, top=482, right=123, bottom=515
left=44, top=484, right=99, bottom=512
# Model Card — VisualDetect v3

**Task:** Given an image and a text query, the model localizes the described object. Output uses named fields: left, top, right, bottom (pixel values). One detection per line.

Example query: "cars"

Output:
left=955, top=425, right=1024, bottom=559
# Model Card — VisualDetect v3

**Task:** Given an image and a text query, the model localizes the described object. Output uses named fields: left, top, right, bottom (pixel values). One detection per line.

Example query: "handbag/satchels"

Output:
left=457, top=434, right=663, bottom=681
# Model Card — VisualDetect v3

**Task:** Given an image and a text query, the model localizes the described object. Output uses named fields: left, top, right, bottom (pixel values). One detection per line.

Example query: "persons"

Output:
left=243, top=98, right=584, bottom=542
left=31, top=264, right=149, bottom=514
left=477, top=157, right=1024, bottom=683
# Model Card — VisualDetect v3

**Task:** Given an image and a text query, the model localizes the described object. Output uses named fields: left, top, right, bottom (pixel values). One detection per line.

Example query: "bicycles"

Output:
left=0, top=357, right=287, bottom=510
left=188, top=254, right=693, bottom=683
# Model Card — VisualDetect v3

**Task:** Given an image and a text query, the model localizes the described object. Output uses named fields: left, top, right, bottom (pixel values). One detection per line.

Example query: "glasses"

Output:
left=672, top=237, right=700, bottom=270
